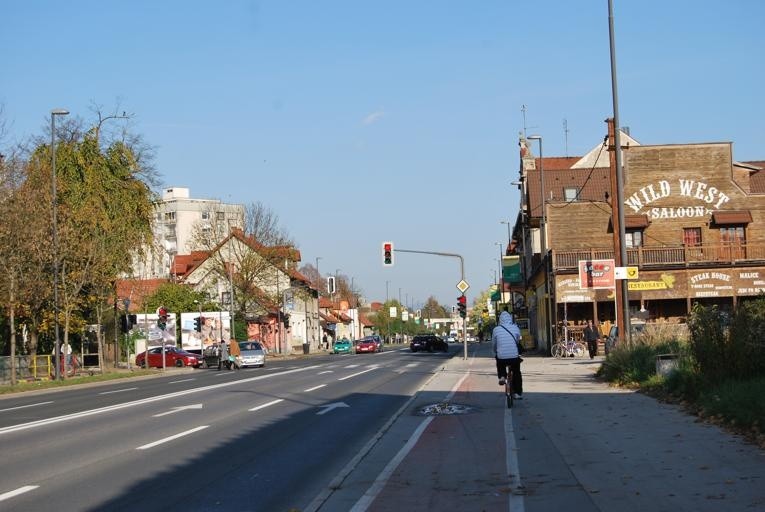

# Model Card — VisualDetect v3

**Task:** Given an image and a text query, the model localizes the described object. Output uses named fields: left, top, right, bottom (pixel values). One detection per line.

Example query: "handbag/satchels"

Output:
left=517, top=343, right=524, bottom=354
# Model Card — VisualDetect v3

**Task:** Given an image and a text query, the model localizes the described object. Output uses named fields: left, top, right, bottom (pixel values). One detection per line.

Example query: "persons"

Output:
left=478, top=330, right=483, bottom=342
left=229, top=337, right=241, bottom=370
left=216, top=340, right=230, bottom=371
left=584, top=320, right=601, bottom=359
left=193, top=318, right=230, bottom=343
left=323, top=334, right=347, bottom=350
left=492, top=311, right=522, bottom=400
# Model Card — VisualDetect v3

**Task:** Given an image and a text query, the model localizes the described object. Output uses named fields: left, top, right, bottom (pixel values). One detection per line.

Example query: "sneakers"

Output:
left=499, top=378, right=505, bottom=385
left=512, top=393, right=522, bottom=399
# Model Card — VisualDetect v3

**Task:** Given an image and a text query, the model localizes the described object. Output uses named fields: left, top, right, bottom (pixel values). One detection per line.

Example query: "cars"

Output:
left=410, top=333, right=448, bottom=352
left=203, top=345, right=234, bottom=369
left=234, top=341, right=265, bottom=367
left=356, top=335, right=384, bottom=354
left=334, top=338, right=353, bottom=354
left=136, top=346, right=204, bottom=369
left=603, top=324, right=647, bottom=358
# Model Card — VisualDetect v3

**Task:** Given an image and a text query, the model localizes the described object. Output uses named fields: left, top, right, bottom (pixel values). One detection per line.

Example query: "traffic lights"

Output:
left=457, top=296, right=466, bottom=318
left=156, top=308, right=167, bottom=330
left=383, top=243, right=394, bottom=265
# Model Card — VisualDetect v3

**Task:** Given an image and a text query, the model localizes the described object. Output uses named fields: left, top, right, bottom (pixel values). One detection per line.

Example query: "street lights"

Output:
left=488, top=258, right=500, bottom=286
left=314, top=256, right=323, bottom=351
left=499, top=220, right=514, bottom=324
left=192, top=299, right=203, bottom=358
left=227, top=217, right=238, bottom=341
left=526, top=133, right=553, bottom=357
left=49, top=107, right=70, bottom=382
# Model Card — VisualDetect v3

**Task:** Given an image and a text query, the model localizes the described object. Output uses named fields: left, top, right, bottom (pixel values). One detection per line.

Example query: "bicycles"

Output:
left=551, top=336, right=587, bottom=360
left=505, top=366, right=513, bottom=409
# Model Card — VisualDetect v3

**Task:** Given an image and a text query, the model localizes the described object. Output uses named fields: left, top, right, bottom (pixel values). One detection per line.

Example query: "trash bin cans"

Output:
left=303, top=344, right=310, bottom=354
